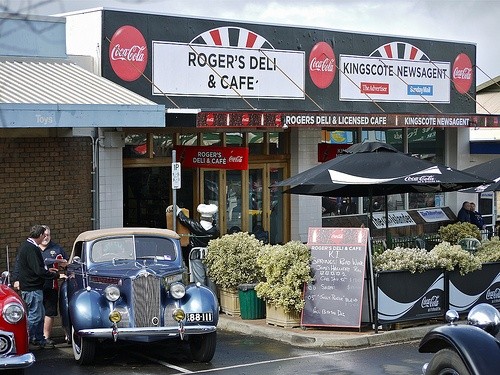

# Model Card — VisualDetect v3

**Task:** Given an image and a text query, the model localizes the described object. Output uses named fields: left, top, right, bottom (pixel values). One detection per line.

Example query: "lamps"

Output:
left=281, top=117, right=288, bottom=128
left=469, top=120, right=479, bottom=130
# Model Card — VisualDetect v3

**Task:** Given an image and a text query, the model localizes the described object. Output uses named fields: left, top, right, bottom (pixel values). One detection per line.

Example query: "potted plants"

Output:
left=205, top=232, right=267, bottom=319
left=256, top=242, right=314, bottom=328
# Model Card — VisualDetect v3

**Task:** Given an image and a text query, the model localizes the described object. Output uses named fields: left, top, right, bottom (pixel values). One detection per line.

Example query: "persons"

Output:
left=458, top=201, right=485, bottom=230
left=13, top=224, right=69, bottom=349
left=167, top=204, right=220, bottom=247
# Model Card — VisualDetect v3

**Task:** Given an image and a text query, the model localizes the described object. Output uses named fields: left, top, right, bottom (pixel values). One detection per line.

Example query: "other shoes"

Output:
left=29, top=337, right=55, bottom=349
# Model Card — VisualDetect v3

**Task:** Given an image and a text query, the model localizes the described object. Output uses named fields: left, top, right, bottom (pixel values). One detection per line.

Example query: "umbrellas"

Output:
left=269, top=138, right=496, bottom=255
left=458, top=157, right=500, bottom=237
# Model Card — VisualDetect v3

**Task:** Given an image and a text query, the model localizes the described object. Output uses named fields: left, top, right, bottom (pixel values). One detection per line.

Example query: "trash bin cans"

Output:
left=237, top=284, right=264, bottom=319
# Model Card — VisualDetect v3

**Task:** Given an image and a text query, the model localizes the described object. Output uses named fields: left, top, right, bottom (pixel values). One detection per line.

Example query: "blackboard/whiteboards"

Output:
left=301, top=227, right=374, bottom=328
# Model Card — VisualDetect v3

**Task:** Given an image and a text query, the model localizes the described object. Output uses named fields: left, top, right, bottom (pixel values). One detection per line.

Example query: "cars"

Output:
left=57, top=225, right=221, bottom=368
left=417, top=302, right=500, bottom=375
left=0, top=269, right=37, bottom=375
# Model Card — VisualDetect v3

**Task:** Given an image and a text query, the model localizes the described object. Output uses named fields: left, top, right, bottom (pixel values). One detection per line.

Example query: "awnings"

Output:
left=0, top=55, right=166, bottom=128
